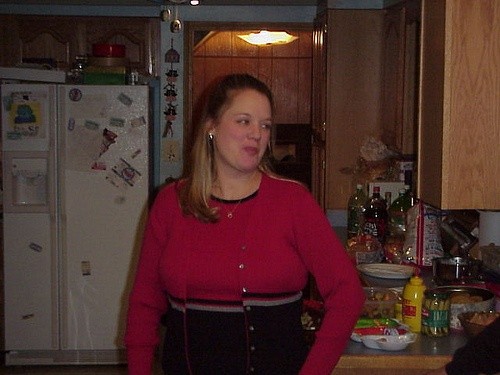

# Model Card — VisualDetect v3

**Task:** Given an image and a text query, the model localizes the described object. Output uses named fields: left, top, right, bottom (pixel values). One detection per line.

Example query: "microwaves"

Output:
left=259, top=123, right=314, bottom=193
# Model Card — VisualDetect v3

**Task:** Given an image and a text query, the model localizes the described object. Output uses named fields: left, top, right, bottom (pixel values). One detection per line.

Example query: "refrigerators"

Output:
left=1, top=83, right=152, bottom=367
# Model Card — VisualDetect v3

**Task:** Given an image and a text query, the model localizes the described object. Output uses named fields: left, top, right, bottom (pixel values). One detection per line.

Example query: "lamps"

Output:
left=236, top=30, right=298, bottom=47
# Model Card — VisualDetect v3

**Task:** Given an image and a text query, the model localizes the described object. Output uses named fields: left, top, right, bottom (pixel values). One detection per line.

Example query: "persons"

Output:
left=428, top=316, right=500, bottom=375
left=125, top=74, right=365, bottom=375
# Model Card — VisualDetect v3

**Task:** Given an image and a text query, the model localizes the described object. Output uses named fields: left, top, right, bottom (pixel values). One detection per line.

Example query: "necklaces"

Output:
left=221, top=199, right=241, bottom=218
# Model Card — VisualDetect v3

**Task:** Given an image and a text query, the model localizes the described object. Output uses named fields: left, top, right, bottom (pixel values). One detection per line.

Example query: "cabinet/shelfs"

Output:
left=311, top=0, right=500, bottom=209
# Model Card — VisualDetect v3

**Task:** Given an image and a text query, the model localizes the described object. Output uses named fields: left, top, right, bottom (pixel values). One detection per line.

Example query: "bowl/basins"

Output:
left=456, top=310, right=500, bottom=336
left=345, top=243, right=381, bottom=263
left=424, top=285, right=496, bottom=332
left=384, top=243, right=405, bottom=263
left=388, top=287, right=404, bottom=320
left=87, top=43, right=127, bottom=67
left=432, top=257, right=482, bottom=287
left=360, top=287, right=396, bottom=317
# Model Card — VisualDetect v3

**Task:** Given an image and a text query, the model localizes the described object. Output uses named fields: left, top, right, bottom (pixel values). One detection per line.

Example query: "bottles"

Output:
left=401, top=276, right=428, bottom=332
left=347, top=183, right=416, bottom=245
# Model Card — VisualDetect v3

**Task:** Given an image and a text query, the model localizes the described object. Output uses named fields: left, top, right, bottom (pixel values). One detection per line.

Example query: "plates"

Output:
left=356, top=263, right=416, bottom=279
left=352, top=318, right=418, bottom=352
